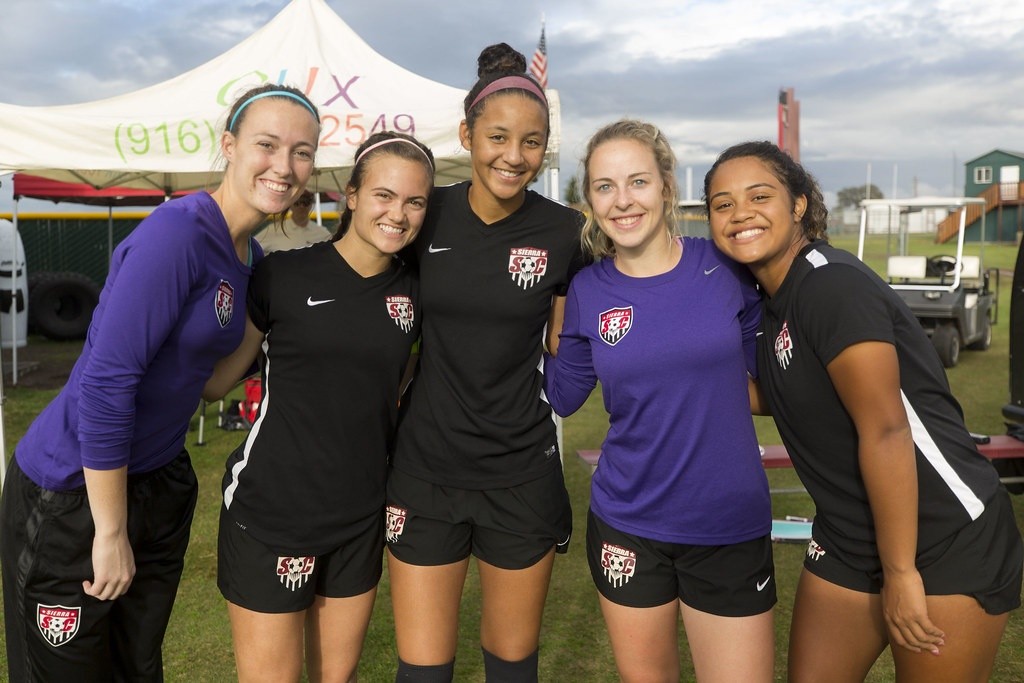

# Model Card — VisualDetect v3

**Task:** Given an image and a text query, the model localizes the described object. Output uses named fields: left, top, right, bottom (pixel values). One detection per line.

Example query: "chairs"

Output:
left=941, top=256, right=982, bottom=294
left=888, top=256, right=928, bottom=289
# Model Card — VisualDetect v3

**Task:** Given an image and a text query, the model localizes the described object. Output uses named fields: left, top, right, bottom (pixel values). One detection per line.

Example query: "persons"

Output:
left=251, top=188, right=333, bottom=257
left=542, top=121, right=779, bottom=683
left=198, top=132, right=435, bottom=683
left=387, top=42, right=594, bottom=683
left=703, top=141, right=1024, bottom=683
left=0, top=85, right=321, bottom=683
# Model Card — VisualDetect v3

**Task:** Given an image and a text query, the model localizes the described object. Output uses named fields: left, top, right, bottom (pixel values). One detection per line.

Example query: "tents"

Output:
left=2, top=0, right=563, bottom=387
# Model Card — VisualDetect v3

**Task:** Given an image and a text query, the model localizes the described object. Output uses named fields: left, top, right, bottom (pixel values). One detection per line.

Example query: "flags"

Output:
left=528, top=27, right=548, bottom=89
